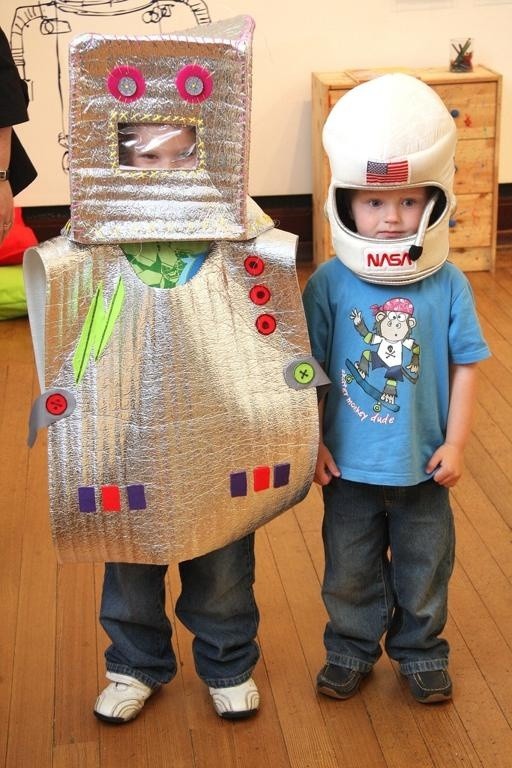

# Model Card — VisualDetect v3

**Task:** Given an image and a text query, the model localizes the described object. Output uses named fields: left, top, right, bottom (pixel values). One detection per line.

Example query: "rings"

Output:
left=3, top=220, right=14, bottom=233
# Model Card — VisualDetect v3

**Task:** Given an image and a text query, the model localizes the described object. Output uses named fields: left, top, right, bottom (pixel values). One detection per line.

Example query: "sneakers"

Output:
left=206, top=675, right=262, bottom=722
left=92, top=670, right=155, bottom=726
left=409, top=662, right=455, bottom=704
left=314, top=661, right=365, bottom=701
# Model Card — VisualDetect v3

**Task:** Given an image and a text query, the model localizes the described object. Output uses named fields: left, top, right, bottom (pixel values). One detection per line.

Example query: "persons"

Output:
left=21, top=12, right=335, bottom=726
left=0, top=22, right=40, bottom=245
left=302, top=67, right=497, bottom=707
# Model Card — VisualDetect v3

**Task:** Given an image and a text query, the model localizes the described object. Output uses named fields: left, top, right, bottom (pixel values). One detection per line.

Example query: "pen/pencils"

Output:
left=451, top=37, right=473, bottom=72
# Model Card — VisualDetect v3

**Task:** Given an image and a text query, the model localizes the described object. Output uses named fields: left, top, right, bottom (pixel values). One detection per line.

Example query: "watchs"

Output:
left=1, top=170, right=9, bottom=182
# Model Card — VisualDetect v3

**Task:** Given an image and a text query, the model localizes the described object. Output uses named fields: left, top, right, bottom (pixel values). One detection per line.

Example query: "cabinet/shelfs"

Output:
left=313, top=64, right=504, bottom=276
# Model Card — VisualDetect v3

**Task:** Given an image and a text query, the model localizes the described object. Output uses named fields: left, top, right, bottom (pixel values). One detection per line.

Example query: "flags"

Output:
left=366, top=159, right=410, bottom=185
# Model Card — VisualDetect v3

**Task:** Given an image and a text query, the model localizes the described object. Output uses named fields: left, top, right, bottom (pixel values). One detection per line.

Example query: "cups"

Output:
left=449, top=38, right=476, bottom=74
